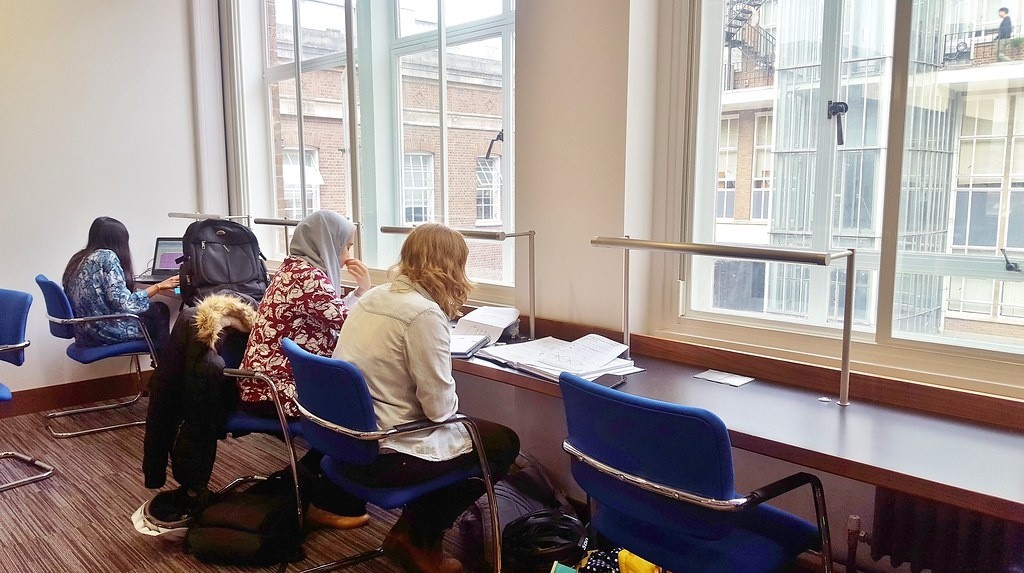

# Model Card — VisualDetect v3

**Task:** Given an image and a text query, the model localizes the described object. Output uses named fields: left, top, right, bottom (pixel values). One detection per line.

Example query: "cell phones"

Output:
left=591, top=373, right=628, bottom=388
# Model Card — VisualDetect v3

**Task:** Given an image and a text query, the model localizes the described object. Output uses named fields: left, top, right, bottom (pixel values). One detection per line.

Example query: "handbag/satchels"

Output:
left=182, top=469, right=310, bottom=566
left=501, top=508, right=587, bottom=573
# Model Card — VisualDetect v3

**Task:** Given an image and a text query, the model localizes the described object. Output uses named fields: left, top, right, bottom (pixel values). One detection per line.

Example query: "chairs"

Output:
left=0, top=288, right=57, bottom=492
left=558, top=369, right=834, bottom=573
left=223, top=330, right=310, bottom=529
left=36, top=273, right=160, bottom=439
left=280, top=337, right=503, bottom=572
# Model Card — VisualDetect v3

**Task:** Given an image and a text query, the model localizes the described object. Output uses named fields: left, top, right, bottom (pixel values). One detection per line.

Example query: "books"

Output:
left=475, top=334, right=646, bottom=384
left=451, top=335, right=490, bottom=359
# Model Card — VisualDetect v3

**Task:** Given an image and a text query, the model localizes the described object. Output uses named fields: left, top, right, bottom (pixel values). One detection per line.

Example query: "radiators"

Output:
left=845, top=484, right=1010, bottom=573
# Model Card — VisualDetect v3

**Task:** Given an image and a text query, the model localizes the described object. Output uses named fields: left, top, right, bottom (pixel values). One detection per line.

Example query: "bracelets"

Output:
left=156, top=284, right=160, bottom=290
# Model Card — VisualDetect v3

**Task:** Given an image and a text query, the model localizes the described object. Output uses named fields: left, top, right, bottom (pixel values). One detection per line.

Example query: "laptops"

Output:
left=133, top=237, right=185, bottom=283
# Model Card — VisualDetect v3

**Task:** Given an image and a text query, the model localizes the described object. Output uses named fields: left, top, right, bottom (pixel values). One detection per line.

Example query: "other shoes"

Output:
left=382, top=503, right=464, bottom=573
left=306, top=503, right=371, bottom=529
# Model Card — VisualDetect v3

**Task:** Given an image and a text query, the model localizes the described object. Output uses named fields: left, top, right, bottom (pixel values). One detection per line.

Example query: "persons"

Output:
left=993, top=7, right=1012, bottom=62
left=332, top=222, right=520, bottom=573
left=236, top=211, right=371, bottom=530
left=61, top=216, right=180, bottom=368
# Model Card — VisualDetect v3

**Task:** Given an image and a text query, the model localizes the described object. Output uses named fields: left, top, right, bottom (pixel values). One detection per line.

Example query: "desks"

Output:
left=132, top=281, right=1024, bottom=527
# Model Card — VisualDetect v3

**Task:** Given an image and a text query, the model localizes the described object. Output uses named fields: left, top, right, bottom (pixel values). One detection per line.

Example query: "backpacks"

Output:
left=175, top=218, right=270, bottom=312
left=458, top=451, right=578, bottom=573
